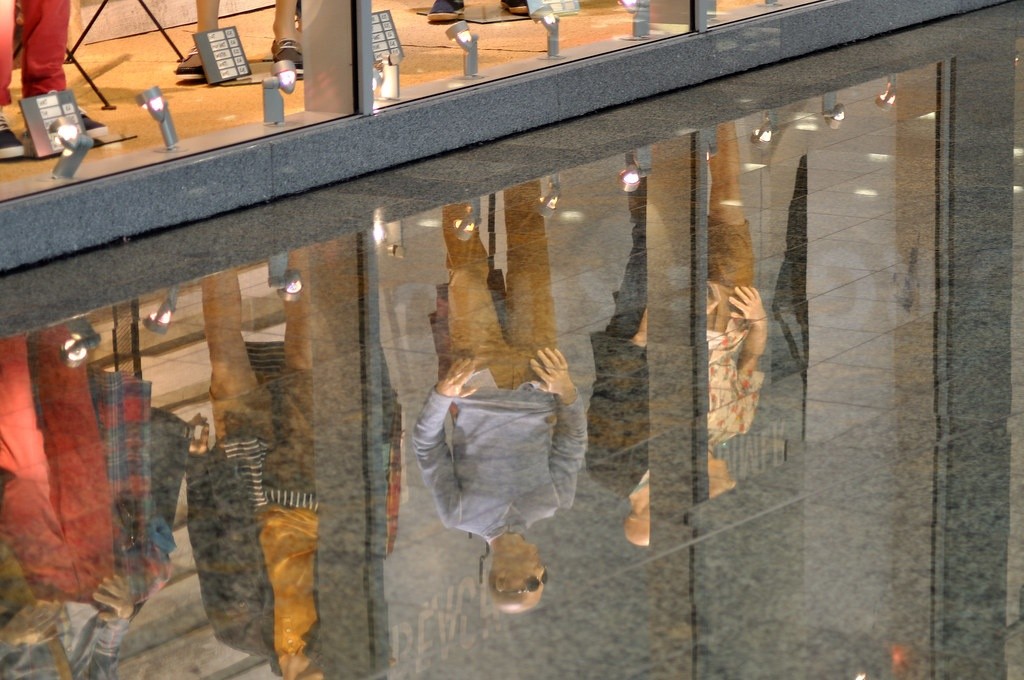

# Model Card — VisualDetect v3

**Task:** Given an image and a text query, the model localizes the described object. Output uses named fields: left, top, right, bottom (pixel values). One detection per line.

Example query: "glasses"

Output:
left=499, top=572, right=549, bottom=594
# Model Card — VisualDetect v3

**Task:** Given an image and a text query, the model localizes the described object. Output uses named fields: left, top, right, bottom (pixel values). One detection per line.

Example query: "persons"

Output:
left=174, top=0, right=307, bottom=79
left=183, top=235, right=403, bottom=680
left=587, top=121, right=767, bottom=547
left=426, top=0, right=528, bottom=23
left=0, top=320, right=195, bottom=680
left=413, top=181, right=589, bottom=614
left=0, top=0, right=115, bottom=160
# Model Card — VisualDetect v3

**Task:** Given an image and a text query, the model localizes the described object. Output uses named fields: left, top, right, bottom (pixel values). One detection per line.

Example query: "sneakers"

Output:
left=271, top=38, right=304, bottom=75
left=175, top=43, right=206, bottom=79
left=49, top=90, right=107, bottom=138
left=0, top=113, right=25, bottom=159
left=500, top=0, right=529, bottom=14
left=427, top=0, right=464, bottom=21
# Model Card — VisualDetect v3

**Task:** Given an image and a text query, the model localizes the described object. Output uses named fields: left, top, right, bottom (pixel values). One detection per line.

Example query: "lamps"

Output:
left=444, top=20, right=482, bottom=80
left=530, top=5, right=562, bottom=58
left=532, top=73, right=898, bottom=220
left=48, top=116, right=95, bottom=180
left=619, top=0, right=650, bottom=39
left=135, top=85, right=180, bottom=152
left=58, top=198, right=478, bottom=370
left=378, top=53, right=404, bottom=100
left=262, top=59, right=298, bottom=128
